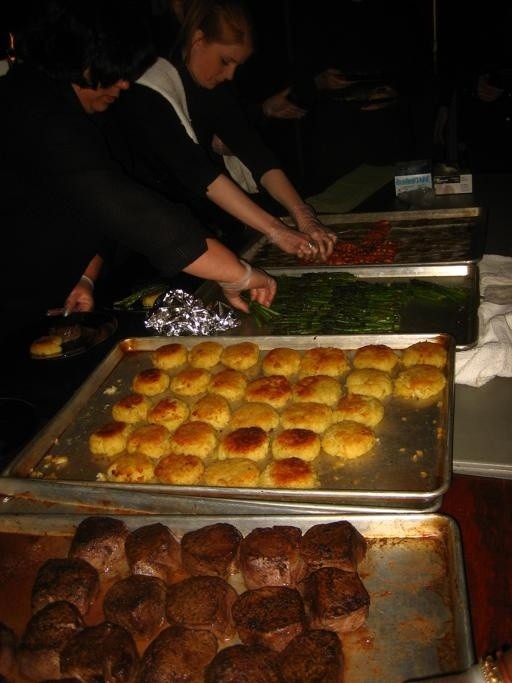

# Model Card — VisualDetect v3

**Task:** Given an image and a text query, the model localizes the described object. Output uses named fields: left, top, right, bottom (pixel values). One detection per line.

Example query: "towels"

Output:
left=220, top=151, right=260, bottom=194
left=137, top=57, right=199, bottom=148
left=450, top=253, right=512, bottom=388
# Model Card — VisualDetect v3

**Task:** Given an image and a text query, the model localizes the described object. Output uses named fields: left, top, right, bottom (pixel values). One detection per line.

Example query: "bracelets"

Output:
left=210, top=258, right=253, bottom=291
left=82, top=274, right=94, bottom=291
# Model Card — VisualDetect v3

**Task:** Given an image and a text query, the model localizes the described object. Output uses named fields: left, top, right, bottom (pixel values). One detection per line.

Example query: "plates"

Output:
left=23, top=309, right=118, bottom=365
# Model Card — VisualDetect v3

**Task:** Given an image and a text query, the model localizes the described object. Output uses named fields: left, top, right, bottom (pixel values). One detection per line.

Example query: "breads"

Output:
left=60, top=618, right=135, bottom=682
left=403, top=341, right=448, bottom=367
left=301, top=347, right=351, bottom=377
left=232, top=584, right=305, bottom=649
left=172, top=420, right=217, bottom=458
left=131, top=367, right=169, bottom=396
left=155, top=453, right=204, bottom=485
left=167, top=575, right=236, bottom=639
left=134, top=623, right=214, bottom=682
left=67, top=513, right=124, bottom=568
left=208, top=368, right=248, bottom=402
left=217, top=426, right=268, bottom=461
left=245, top=374, right=292, bottom=407
left=150, top=341, right=188, bottom=370
left=333, top=394, right=384, bottom=427
left=30, top=334, right=61, bottom=355
left=292, top=375, right=342, bottom=406
left=222, top=341, right=258, bottom=371
left=111, top=392, right=151, bottom=422
left=108, top=450, right=153, bottom=483
left=147, top=396, right=189, bottom=431
left=28, top=555, right=99, bottom=614
left=188, top=341, right=222, bottom=368
left=18, top=599, right=81, bottom=681
left=232, top=402, right=279, bottom=431
left=127, top=422, right=172, bottom=457
left=190, top=393, right=232, bottom=429
left=278, top=627, right=344, bottom=682
left=353, top=343, right=397, bottom=370
left=89, top=420, right=135, bottom=455
left=100, top=572, right=165, bottom=633
left=182, top=520, right=242, bottom=578
left=272, top=427, right=321, bottom=461
left=304, top=520, right=368, bottom=568
left=243, top=523, right=301, bottom=583
left=206, top=640, right=278, bottom=682
left=299, top=565, right=368, bottom=630
left=260, top=457, right=321, bottom=488
left=395, top=364, right=447, bottom=399
left=281, top=402, right=333, bottom=433
left=261, top=345, right=300, bottom=375
left=322, top=420, right=376, bottom=458
left=170, top=367, right=213, bottom=395
left=204, top=457, right=259, bottom=486
left=124, top=523, right=181, bottom=576
left=345, top=367, right=392, bottom=400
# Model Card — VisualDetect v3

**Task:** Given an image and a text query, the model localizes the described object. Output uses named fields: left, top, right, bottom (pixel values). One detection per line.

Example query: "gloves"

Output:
left=217, top=259, right=277, bottom=311
left=265, top=227, right=318, bottom=261
left=47, top=275, right=95, bottom=317
left=289, top=204, right=338, bottom=262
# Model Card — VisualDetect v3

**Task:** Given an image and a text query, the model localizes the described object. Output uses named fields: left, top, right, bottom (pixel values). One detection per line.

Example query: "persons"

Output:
left=243, top=86, right=309, bottom=121
left=107, top=6, right=340, bottom=261
left=3, top=10, right=276, bottom=318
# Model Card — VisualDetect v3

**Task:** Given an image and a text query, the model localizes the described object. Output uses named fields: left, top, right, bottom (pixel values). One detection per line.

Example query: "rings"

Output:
left=308, top=240, right=313, bottom=248
